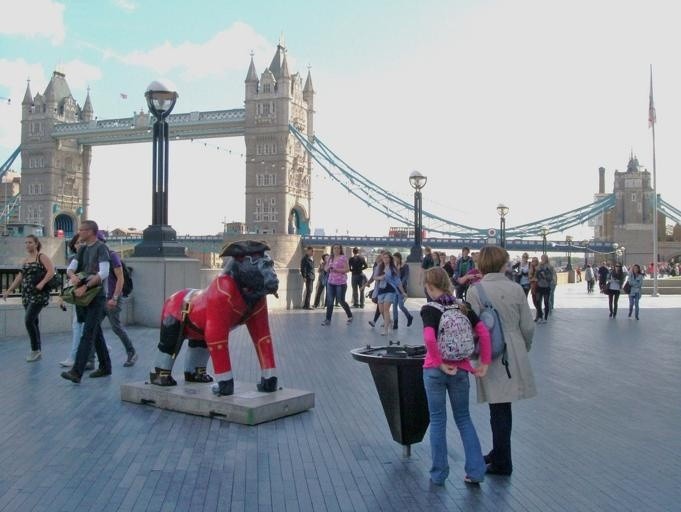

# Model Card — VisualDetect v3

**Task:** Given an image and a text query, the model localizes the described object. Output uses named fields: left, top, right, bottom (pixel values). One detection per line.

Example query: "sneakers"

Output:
left=368, top=318, right=413, bottom=336
left=321, top=319, right=330, bottom=326
left=346, top=317, right=353, bottom=327
left=485, top=465, right=512, bottom=475
left=464, top=474, right=484, bottom=482
left=58, top=358, right=112, bottom=383
left=124, top=353, right=137, bottom=367
left=27, top=351, right=41, bottom=361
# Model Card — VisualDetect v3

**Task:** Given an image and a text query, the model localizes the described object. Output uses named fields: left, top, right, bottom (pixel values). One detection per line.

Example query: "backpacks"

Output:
left=474, top=282, right=504, bottom=358
left=109, top=251, right=133, bottom=297
left=423, top=299, right=475, bottom=361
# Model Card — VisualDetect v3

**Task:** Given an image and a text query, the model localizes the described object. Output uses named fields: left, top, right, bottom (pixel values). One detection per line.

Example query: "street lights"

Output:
left=613, top=242, right=619, bottom=261
left=495, top=202, right=510, bottom=250
left=541, top=226, right=550, bottom=254
left=621, top=246, right=626, bottom=264
left=566, top=233, right=574, bottom=271
left=583, top=238, right=590, bottom=270
left=132, top=78, right=188, bottom=258
left=406, top=170, right=428, bottom=262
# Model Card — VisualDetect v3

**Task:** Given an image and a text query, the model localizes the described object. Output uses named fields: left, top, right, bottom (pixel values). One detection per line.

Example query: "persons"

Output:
left=420, top=247, right=557, bottom=485
left=3, top=220, right=136, bottom=384
left=576, top=262, right=681, bottom=321
left=300, top=243, right=413, bottom=338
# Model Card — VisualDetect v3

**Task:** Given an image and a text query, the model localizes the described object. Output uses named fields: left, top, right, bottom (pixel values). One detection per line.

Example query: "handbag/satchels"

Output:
left=59, top=272, right=101, bottom=307
left=37, top=254, right=62, bottom=289
left=623, top=282, right=631, bottom=294
left=603, top=283, right=609, bottom=294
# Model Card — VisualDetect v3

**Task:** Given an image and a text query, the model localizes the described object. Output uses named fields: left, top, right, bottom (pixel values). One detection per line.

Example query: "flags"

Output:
left=648, top=87, right=657, bottom=129
